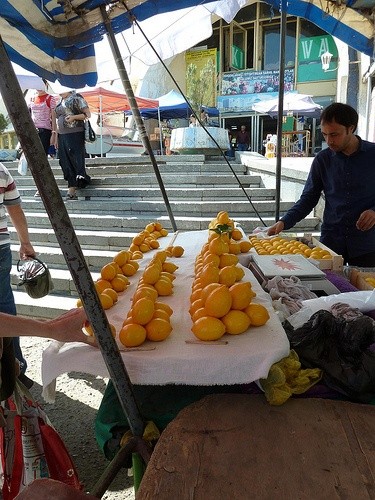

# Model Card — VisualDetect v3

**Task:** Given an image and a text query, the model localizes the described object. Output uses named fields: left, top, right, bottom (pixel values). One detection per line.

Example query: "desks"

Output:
left=282, top=131, right=310, bottom=156
left=37, top=222, right=292, bottom=499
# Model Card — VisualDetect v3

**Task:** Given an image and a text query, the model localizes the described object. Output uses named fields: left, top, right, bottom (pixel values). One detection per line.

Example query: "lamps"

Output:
left=320, top=50, right=340, bottom=72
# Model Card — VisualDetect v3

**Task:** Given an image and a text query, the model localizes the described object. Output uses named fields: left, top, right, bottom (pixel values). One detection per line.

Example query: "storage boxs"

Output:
left=247, top=232, right=344, bottom=274
left=350, top=268, right=375, bottom=291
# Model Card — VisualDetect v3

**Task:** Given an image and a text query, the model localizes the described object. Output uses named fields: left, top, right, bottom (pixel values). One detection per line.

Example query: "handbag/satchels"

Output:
left=17, top=255, right=54, bottom=299
left=16, top=141, right=27, bottom=175
left=0, top=378, right=85, bottom=500
left=85, top=120, right=95, bottom=143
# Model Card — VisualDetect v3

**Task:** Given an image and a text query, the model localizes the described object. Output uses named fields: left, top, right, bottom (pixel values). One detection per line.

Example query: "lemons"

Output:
left=76, top=211, right=375, bottom=348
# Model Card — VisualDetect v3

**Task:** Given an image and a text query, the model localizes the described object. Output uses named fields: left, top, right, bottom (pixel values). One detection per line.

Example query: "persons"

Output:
left=24, top=88, right=57, bottom=198
left=0, top=306, right=100, bottom=350
left=263, top=101, right=375, bottom=270
left=0, top=161, right=36, bottom=374
left=54, top=90, right=91, bottom=198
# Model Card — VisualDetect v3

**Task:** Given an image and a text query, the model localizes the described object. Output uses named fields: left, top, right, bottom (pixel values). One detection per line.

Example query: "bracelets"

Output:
left=51, top=131, right=57, bottom=135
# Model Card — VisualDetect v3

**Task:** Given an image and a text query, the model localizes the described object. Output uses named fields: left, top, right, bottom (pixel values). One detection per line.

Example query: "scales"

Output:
left=247, top=254, right=341, bottom=299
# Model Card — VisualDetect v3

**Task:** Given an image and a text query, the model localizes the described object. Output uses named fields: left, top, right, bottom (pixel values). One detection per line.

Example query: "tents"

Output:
left=56, top=88, right=220, bottom=160
left=0, top=0, right=375, bottom=445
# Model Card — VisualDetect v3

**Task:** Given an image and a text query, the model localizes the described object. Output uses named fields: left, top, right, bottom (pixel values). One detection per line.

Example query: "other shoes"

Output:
left=34, top=190, right=40, bottom=197
left=66, top=193, right=78, bottom=200
